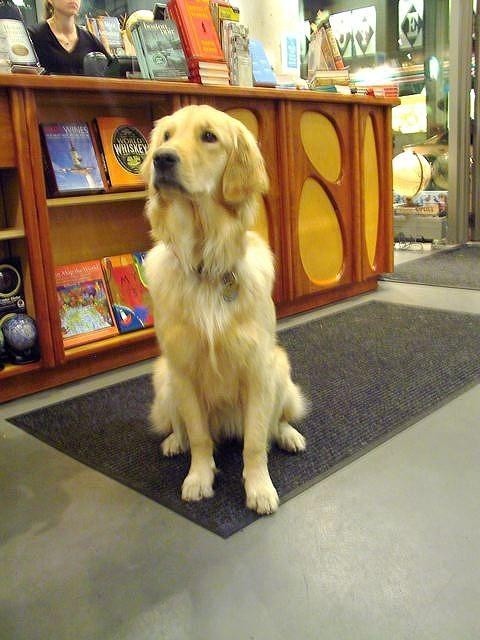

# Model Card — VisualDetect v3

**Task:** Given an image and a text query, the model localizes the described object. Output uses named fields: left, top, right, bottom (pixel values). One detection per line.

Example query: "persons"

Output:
left=27, top=1, right=115, bottom=78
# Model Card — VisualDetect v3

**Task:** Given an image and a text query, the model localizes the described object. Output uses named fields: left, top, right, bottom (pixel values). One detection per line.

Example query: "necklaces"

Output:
left=52, top=17, right=69, bottom=46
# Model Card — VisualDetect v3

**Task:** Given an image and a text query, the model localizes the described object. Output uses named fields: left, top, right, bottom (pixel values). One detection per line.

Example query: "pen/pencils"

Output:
left=118, top=12, right=127, bottom=29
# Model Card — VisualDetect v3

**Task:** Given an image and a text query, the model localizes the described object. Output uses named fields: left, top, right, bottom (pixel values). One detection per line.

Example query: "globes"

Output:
left=433, top=150, right=449, bottom=193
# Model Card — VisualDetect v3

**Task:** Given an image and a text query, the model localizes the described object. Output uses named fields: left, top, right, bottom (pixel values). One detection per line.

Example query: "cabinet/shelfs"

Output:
left=0, top=74, right=400, bottom=407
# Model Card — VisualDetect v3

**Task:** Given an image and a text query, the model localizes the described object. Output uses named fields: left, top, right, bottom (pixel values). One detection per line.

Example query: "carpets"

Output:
left=376, top=243, right=479, bottom=295
left=5, top=300, right=480, bottom=540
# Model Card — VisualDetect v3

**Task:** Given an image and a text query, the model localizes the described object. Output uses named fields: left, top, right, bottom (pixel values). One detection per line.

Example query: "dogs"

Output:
left=136, top=102, right=314, bottom=518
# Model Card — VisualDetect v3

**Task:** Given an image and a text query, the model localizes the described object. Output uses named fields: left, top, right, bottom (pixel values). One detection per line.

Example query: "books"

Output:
left=53, top=258, right=121, bottom=349
left=85, top=121, right=111, bottom=195
left=309, top=17, right=400, bottom=98
left=95, top=116, right=156, bottom=193
left=0, top=253, right=27, bottom=326
left=86, top=0, right=280, bottom=89
left=38, top=120, right=104, bottom=197
left=0, top=0, right=40, bottom=69
left=102, top=251, right=155, bottom=334
